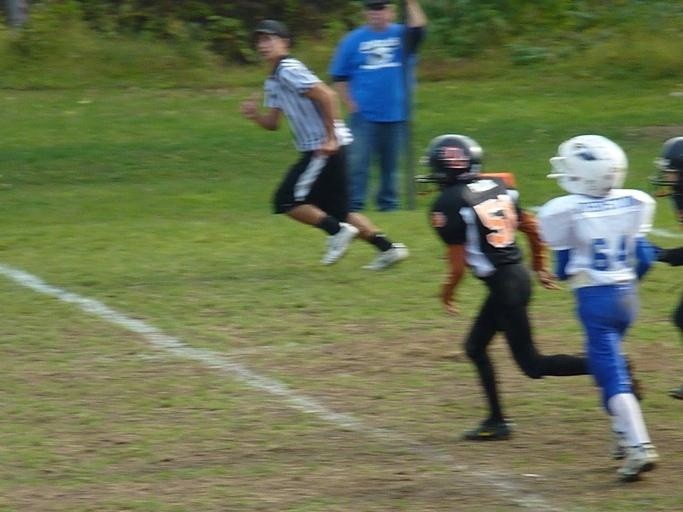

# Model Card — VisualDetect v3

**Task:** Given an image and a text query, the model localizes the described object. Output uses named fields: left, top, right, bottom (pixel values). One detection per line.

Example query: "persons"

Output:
left=412, top=133, right=646, bottom=443
left=647, top=136, right=682, bottom=400
left=324, top=1, right=429, bottom=214
left=238, top=18, right=411, bottom=273
left=534, top=132, right=661, bottom=480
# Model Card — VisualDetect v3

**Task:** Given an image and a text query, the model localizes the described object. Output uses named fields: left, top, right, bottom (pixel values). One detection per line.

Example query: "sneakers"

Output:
left=668, top=385, right=683, bottom=399
left=464, top=418, right=508, bottom=440
left=363, top=241, right=409, bottom=270
left=610, top=436, right=659, bottom=481
left=619, top=351, right=647, bottom=401
left=319, top=221, right=361, bottom=266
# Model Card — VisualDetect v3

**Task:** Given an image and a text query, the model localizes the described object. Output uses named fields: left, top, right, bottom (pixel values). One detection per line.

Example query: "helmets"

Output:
left=413, top=134, right=485, bottom=191
left=545, top=134, right=629, bottom=198
left=646, top=136, right=682, bottom=198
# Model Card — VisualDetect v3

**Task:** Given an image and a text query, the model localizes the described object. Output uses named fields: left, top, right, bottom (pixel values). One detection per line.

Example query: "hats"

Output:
left=249, top=20, right=289, bottom=37
